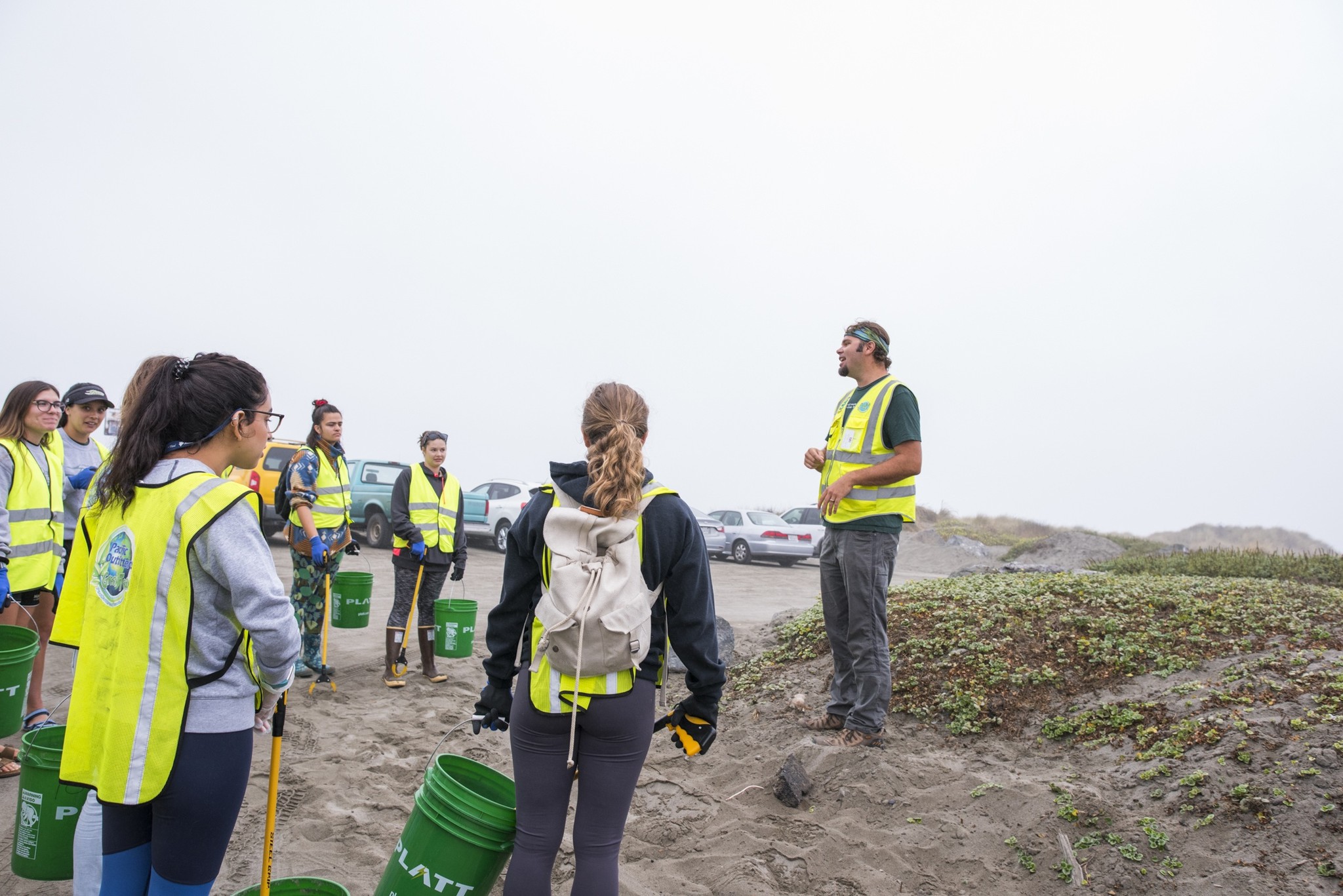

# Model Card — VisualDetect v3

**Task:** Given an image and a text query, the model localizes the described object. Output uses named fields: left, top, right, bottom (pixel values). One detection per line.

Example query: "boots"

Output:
left=288, top=634, right=313, bottom=677
left=381, top=626, right=406, bottom=687
left=302, top=632, right=336, bottom=674
left=417, top=625, right=449, bottom=682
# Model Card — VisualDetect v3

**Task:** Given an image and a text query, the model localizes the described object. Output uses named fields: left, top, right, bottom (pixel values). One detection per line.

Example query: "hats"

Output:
left=64, top=386, right=116, bottom=409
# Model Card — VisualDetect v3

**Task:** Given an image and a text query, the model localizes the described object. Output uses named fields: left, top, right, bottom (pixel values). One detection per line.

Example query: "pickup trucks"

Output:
left=341, top=459, right=493, bottom=547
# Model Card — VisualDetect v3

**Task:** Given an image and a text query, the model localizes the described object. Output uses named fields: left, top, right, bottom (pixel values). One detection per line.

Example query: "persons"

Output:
left=803, top=321, right=923, bottom=748
left=282, top=399, right=361, bottom=678
left=473, top=381, right=727, bottom=896
left=100, top=348, right=301, bottom=895
left=0, top=353, right=182, bottom=894
left=381, top=431, right=468, bottom=686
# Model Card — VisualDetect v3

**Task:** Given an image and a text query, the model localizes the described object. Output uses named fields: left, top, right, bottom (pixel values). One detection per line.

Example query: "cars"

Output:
left=690, top=507, right=727, bottom=556
left=710, top=509, right=815, bottom=565
left=470, top=479, right=540, bottom=554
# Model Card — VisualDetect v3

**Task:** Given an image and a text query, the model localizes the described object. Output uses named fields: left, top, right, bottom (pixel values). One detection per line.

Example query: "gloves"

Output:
left=0, top=567, right=12, bottom=614
left=51, top=573, right=65, bottom=614
left=450, top=567, right=464, bottom=581
left=671, top=697, right=718, bottom=757
left=68, top=466, right=97, bottom=490
left=309, top=535, right=332, bottom=568
left=345, top=539, right=360, bottom=557
left=410, top=541, right=424, bottom=561
left=471, top=681, right=513, bottom=735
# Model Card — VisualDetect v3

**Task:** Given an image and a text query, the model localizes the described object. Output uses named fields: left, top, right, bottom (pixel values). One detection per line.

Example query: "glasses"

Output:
left=230, top=407, right=285, bottom=433
left=423, top=433, right=448, bottom=446
left=32, top=399, right=67, bottom=414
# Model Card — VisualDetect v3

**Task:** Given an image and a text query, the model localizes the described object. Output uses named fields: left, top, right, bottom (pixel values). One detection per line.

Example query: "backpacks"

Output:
left=528, top=481, right=665, bottom=680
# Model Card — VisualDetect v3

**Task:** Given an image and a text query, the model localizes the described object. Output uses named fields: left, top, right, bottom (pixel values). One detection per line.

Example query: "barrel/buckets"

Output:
left=373, top=714, right=518, bottom=896
left=229, top=875, right=350, bottom=896
left=0, top=593, right=42, bottom=740
left=433, top=575, right=477, bottom=658
left=332, top=551, right=374, bottom=628
left=11, top=694, right=92, bottom=880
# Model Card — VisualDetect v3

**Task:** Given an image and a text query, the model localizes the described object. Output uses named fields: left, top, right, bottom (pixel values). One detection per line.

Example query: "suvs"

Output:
left=227, top=439, right=309, bottom=539
left=782, top=502, right=829, bottom=550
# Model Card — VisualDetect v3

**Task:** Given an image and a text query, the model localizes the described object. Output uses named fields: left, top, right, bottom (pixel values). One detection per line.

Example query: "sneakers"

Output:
left=798, top=712, right=846, bottom=731
left=811, top=726, right=885, bottom=749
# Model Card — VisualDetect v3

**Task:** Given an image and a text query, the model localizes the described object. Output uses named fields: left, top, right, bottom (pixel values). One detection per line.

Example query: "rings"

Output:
left=830, top=499, right=834, bottom=502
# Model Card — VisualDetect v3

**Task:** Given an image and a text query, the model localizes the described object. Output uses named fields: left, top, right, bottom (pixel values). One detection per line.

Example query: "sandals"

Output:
left=22, top=708, right=58, bottom=732
left=0, top=745, right=21, bottom=778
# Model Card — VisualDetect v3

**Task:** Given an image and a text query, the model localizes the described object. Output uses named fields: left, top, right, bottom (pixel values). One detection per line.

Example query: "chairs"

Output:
left=497, top=491, right=510, bottom=499
left=366, top=473, right=377, bottom=482
